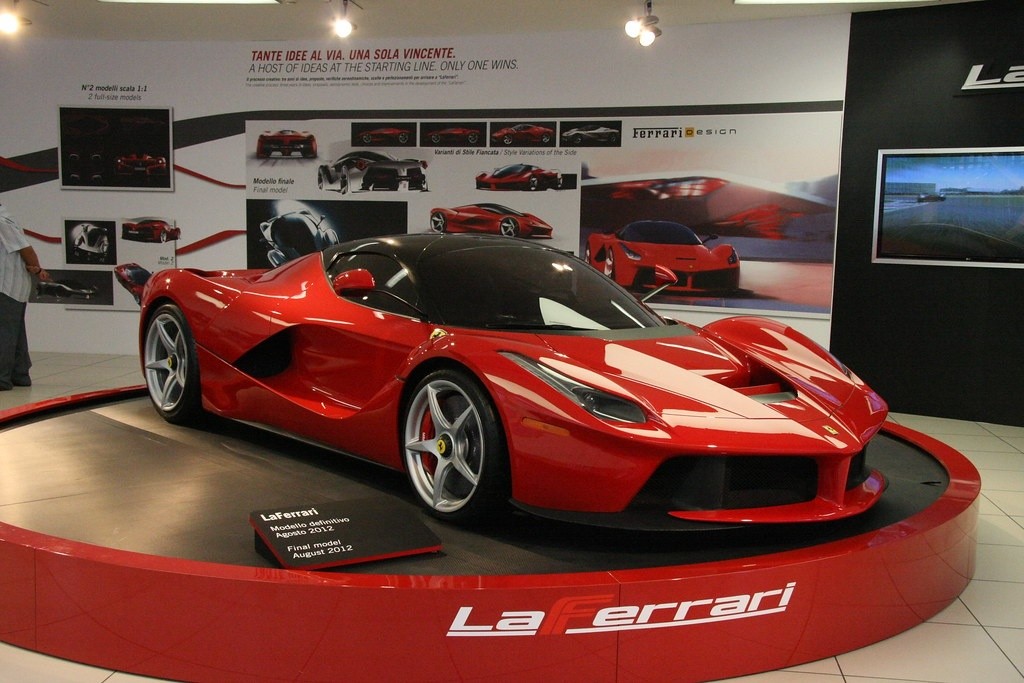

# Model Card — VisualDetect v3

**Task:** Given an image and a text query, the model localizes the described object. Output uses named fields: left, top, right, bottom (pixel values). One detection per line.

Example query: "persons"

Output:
left=1, top=203, right=56, bottom=392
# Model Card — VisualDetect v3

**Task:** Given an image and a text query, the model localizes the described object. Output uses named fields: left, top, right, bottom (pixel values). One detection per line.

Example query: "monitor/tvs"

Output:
left=869, top=146, right=1024, bottom=269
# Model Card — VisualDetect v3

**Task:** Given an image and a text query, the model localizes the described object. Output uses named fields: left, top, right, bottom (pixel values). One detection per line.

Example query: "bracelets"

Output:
left=36, top=269, right=42, bottom=275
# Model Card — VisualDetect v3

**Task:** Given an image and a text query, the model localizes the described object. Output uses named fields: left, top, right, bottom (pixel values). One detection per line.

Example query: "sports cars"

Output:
left=475, top=162, right=564, bottom=193
left=581, top=221, right=741, bottom=296
left=255, top=129, right=318, bottom=159
left=38, top=277, right=100, bottom=299
left=318, top=149, right=430, bottom=195
left=494, top=124, right=554, bottom=146
left=918, top=192, right=946, bottom=203
left=115, top=153, right=167, bottom=182
left=359, top=127, right=410, bottom=145
left=113, top=262, right=152, bottom=306
left=122, top=219, right=181, bottom=243
left=427, top=127, right=480, bottom=145
left=136, top=231, right=889, bottom=529
left=429, top=202, right=552, bottom=239
left=562, top=127, right=620, bottom=146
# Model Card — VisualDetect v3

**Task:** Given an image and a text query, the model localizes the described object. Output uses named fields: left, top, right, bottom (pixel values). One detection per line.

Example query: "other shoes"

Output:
left=0, top=387, right=12, bottom=391
left=13, top=383, right=30, bottom=386
